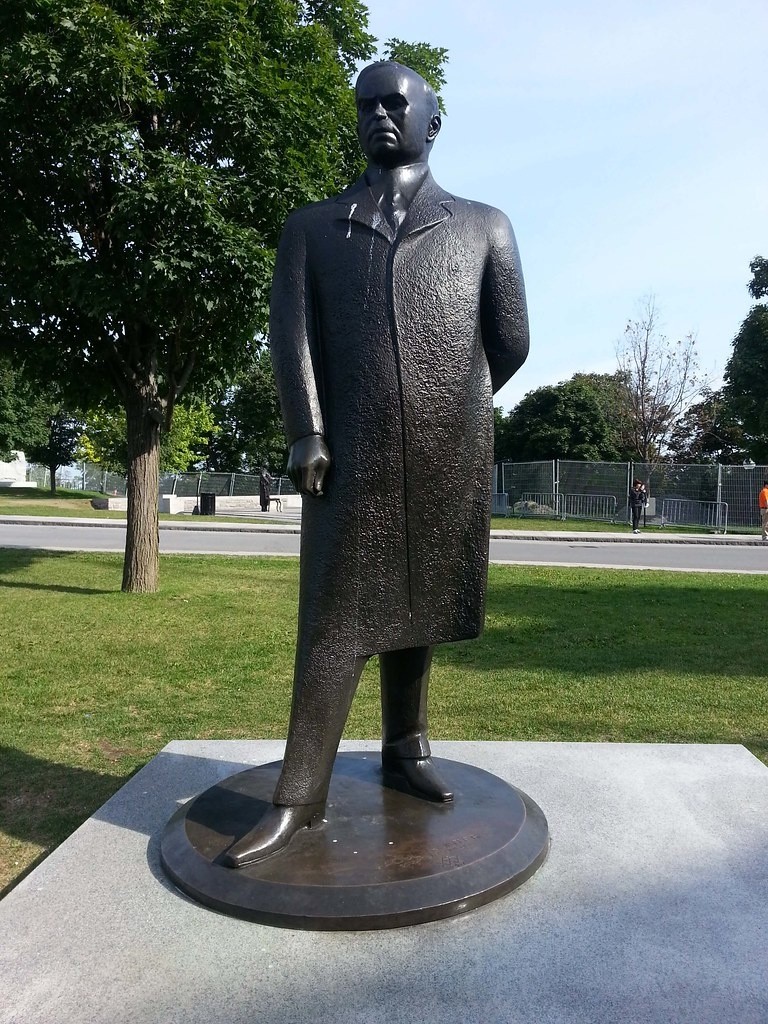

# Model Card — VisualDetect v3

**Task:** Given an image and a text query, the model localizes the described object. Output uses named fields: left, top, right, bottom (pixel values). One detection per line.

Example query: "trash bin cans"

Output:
left=200, top=492, right=215, bottom=515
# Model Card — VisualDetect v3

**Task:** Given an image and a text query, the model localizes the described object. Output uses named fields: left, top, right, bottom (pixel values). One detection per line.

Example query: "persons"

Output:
left=759, top=480, right=768, bottom=541
left=630, top=479, right=646, bottom=533
left=222, top=62, right=529, bottom=866
left=258, top=466, right=272, bottom=512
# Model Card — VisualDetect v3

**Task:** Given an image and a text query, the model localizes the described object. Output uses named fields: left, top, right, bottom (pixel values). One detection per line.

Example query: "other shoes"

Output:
left=634, top=529, right=640, bottom=534
